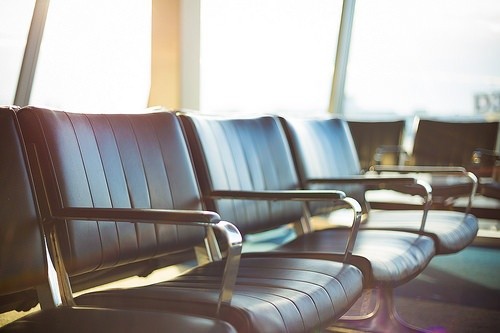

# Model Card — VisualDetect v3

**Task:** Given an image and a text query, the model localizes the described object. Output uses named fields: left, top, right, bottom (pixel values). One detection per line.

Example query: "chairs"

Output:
left=0, top=106, right=499, bottom=333
